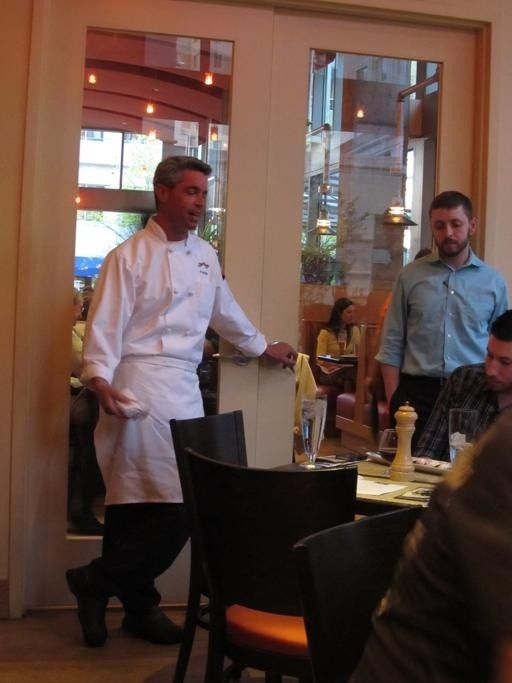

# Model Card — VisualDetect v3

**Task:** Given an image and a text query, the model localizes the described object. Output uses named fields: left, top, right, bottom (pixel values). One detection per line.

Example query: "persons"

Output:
left=363, top=246, right=432, bottom=388
left=347, top=405, right=511, bottom=681
left=376, top=190, right=508, bottom=455
left=63, top=155, right=299, bottom=649
left=410, top=308, right=511, bottom=468
left=315, top=295, right=360, bottom=436
left=68, top=286, right=220, bottom=534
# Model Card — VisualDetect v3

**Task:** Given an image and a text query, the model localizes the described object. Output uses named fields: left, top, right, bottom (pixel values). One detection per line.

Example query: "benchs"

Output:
left=295, top=317, right=392, bottom=457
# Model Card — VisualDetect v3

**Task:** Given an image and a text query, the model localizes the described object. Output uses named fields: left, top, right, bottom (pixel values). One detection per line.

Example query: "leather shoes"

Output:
left=119, top=606, right=187, bottom=645
left=63, top=562, right=109, bottom=649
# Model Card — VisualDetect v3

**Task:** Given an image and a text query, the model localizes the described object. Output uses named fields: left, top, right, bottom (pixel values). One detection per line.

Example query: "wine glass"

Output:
left=299, top=391, right=329, bottom=468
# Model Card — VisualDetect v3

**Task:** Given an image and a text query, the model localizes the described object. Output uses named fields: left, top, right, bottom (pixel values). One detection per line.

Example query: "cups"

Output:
left=379, top=428, right=398, bottom=465
left=447, top=408, right=479, bottom=462
left=338, top=336, right=345, bottom=354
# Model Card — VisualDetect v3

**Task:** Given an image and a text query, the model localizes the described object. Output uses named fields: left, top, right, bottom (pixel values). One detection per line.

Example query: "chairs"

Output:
left=292, top=506, right=423, bottom=683
left=182, top=447, right=359, bottom=682
left=168, top=410, right=250, bottom=683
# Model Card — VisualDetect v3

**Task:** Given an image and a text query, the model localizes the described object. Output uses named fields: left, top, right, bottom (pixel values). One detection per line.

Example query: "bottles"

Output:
left=386, top=398, right=420, bottom=483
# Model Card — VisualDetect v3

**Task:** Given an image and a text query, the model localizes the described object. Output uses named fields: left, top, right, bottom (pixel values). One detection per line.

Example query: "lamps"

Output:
left=305, top=182, right=341, bottom=235
left=378, top=193, right=418, bottom=226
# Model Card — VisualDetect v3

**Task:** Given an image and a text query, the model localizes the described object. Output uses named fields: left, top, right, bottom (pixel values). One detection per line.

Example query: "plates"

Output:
left=364, top=450, right=448, bottom=475
